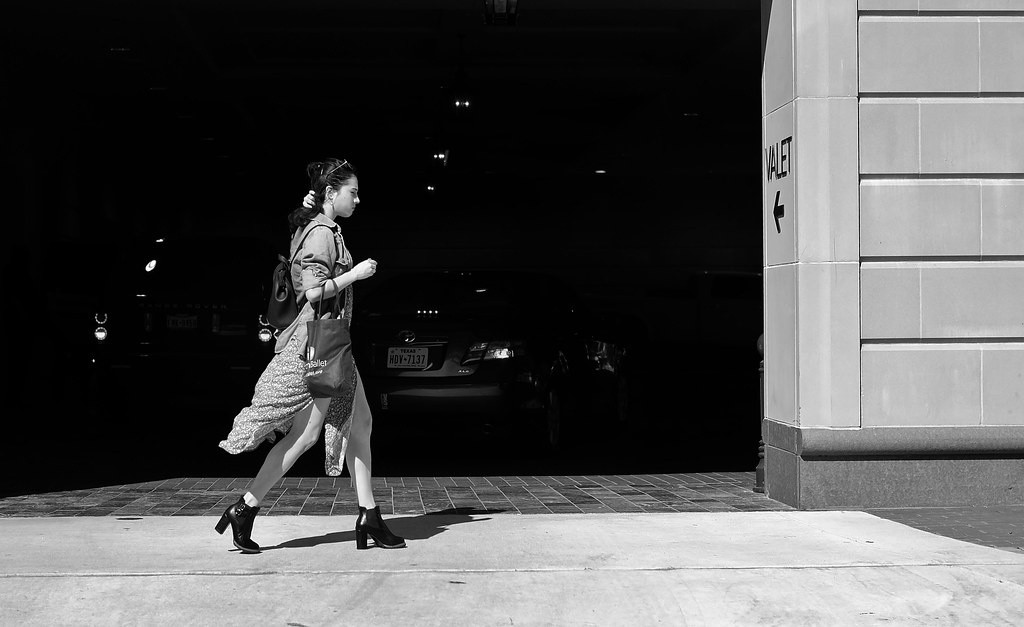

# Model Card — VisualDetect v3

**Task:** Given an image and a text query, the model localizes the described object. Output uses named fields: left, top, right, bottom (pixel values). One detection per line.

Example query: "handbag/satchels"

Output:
left=305, top=278, right=355, bottom=397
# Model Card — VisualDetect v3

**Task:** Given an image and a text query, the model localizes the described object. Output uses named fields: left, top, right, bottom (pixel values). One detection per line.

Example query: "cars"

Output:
left=590, top=267, right=763, bottom=352
left=354, top=268, right=632, bottom=443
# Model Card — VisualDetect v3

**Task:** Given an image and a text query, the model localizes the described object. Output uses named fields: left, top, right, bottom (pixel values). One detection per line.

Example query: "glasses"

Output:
left=327, top=158, right=353, bottom=176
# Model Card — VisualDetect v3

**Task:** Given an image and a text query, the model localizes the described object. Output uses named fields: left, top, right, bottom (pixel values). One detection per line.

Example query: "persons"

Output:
left=214, top=156, right=406, bottom=555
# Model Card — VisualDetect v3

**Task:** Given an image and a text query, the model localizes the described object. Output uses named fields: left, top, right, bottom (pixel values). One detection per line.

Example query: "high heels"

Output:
left=215, top=495, right=260, bottom=552
left=355, top=506, right=406, bottom=550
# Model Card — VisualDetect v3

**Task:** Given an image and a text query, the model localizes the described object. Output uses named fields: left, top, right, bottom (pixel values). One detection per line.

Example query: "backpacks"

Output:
left=269, top=224, right=339, bottom=330
